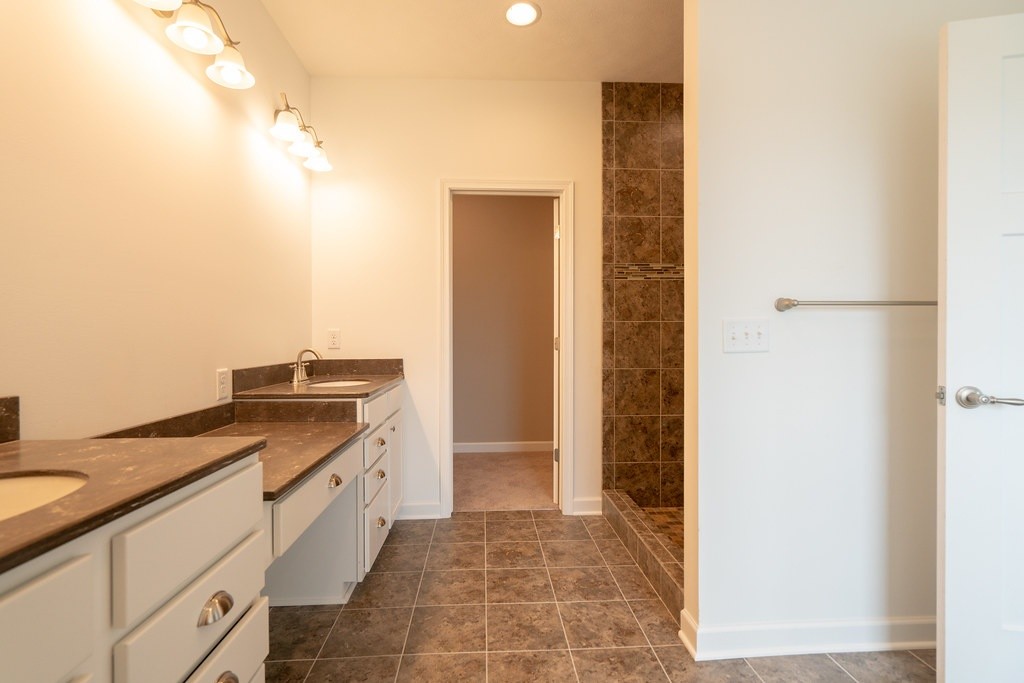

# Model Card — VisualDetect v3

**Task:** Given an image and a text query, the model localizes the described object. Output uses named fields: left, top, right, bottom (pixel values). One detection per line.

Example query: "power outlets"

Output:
left=327, top=328, right=341, bottom=349
left=215, top=367, right=229, bottom=401
left=722, top=318, right=771, bottom=354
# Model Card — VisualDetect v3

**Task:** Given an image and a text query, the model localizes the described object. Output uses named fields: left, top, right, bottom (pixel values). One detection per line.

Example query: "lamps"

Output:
left=132, top=0, right=255, bottom=90
left=271, top=92, right=333, bottom=173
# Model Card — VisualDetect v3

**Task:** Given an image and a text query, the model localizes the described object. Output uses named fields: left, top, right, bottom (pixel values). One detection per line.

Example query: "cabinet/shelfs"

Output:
left=235, top=391, right=390, bottom=605
left=0, top=451, right=270, bottom=683
left=384, top=381, right=405, bottom=529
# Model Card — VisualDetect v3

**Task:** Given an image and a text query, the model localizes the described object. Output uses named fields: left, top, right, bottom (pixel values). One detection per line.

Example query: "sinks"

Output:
left=0, top=470, right=89, bottom=522
left=306, top=379, right=372, bottom=387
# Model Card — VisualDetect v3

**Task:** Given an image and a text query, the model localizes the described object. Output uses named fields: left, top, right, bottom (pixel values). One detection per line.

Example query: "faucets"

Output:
left=297, top=349, right=322, bottom=381
